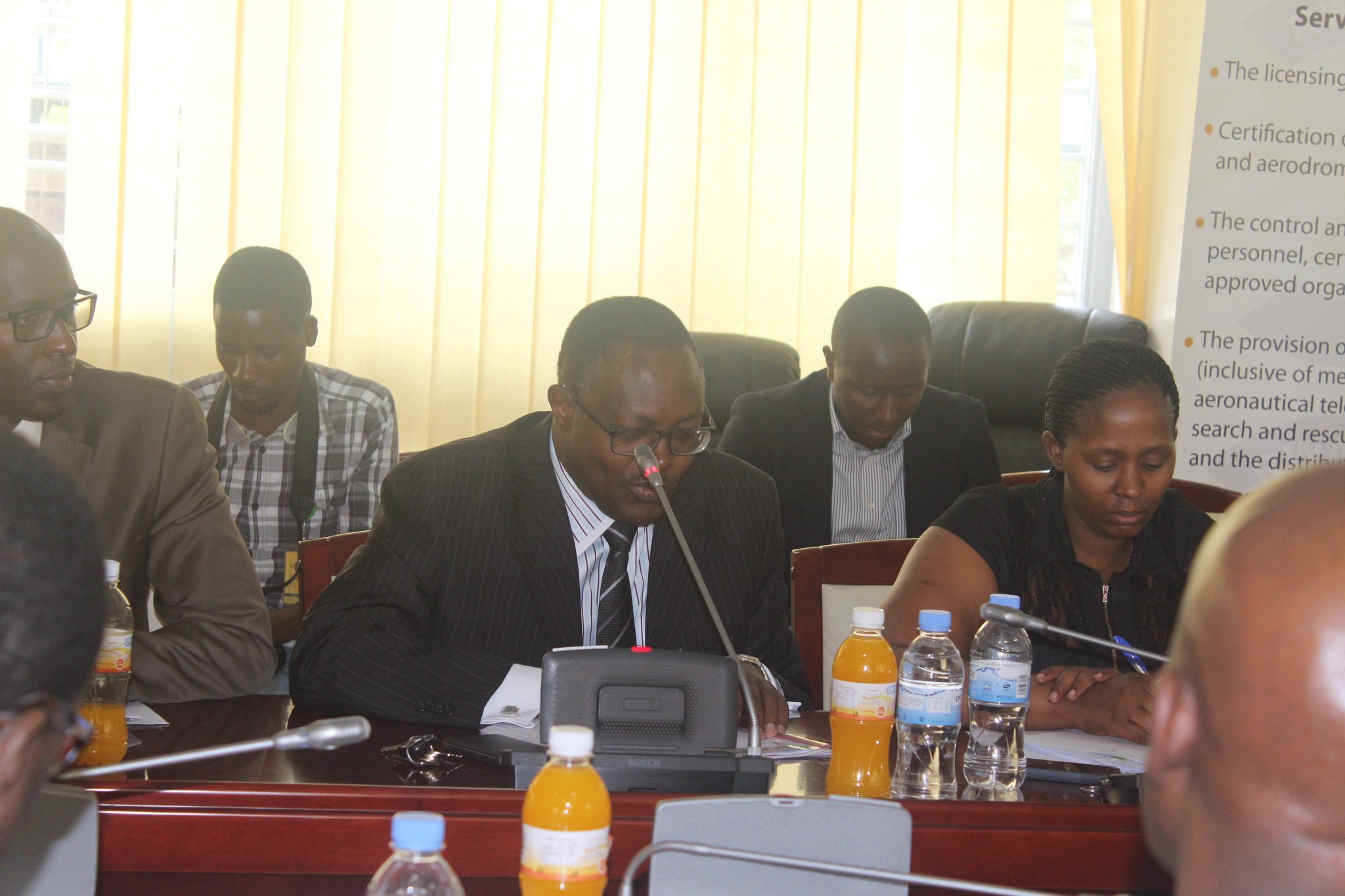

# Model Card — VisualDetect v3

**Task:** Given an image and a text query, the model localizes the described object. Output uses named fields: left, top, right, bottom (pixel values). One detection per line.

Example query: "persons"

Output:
left=715, top=288, right=1008, bottom=632
left=882, top=339, right=1223, bottom=744
left=296, top=297, right=808, bottom=740
left=1, top=207, right=401, bottom=843
left=1139, top=464, right=1345, bottom=896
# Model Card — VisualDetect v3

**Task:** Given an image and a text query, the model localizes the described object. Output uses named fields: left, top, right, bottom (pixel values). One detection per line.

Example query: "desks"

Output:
left=46, top=694, right=1163, bottom=896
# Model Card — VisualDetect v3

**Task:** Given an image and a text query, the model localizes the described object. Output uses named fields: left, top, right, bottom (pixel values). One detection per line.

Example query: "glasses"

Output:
left=0, top=289, right=97, bottom=342
left=562, top=386, right=718, bottom=456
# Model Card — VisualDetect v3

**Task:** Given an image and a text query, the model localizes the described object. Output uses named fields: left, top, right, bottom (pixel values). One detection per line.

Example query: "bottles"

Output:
left=519, top=725, right=615, bottom=896
left=824, top=606, right=899, bottom=798
left=74, top=559, right=134, bottom=765
left=364, top=810, right=466, bottom=896
left=962, top=593, right=1033, bottom=790
left=890, top=609, right=965, bottom=801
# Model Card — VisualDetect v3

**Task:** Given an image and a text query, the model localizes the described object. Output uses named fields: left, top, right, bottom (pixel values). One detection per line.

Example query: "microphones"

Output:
left=56, top=715, right=372, bottom=781
left=980, top=603, right=1168, bottom=661
left=632, top=443, right=761, bottom=755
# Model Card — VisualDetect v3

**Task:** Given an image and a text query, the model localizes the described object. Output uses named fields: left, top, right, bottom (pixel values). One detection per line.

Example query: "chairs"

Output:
left=685, top=328, right=803, bottom=437
left=905, top=298, right=1150, bottom=469
left=787, top=538, right=921, bottom=699
left=1000, top=463, right=1242, bottom=524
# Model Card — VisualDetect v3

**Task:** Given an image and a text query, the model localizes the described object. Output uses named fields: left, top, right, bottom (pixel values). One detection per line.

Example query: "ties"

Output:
left=596, top=521, right=639, bottom=649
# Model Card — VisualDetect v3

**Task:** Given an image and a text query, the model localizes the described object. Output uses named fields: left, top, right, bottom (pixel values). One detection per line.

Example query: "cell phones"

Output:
left=1024, top=758, right=1120, bottom=785
left=442, top=733, right=546, bottom=768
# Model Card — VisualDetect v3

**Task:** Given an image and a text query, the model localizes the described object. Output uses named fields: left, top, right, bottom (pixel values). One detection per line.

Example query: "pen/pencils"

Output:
left=1115, top=634, right=1149, bottom=675
